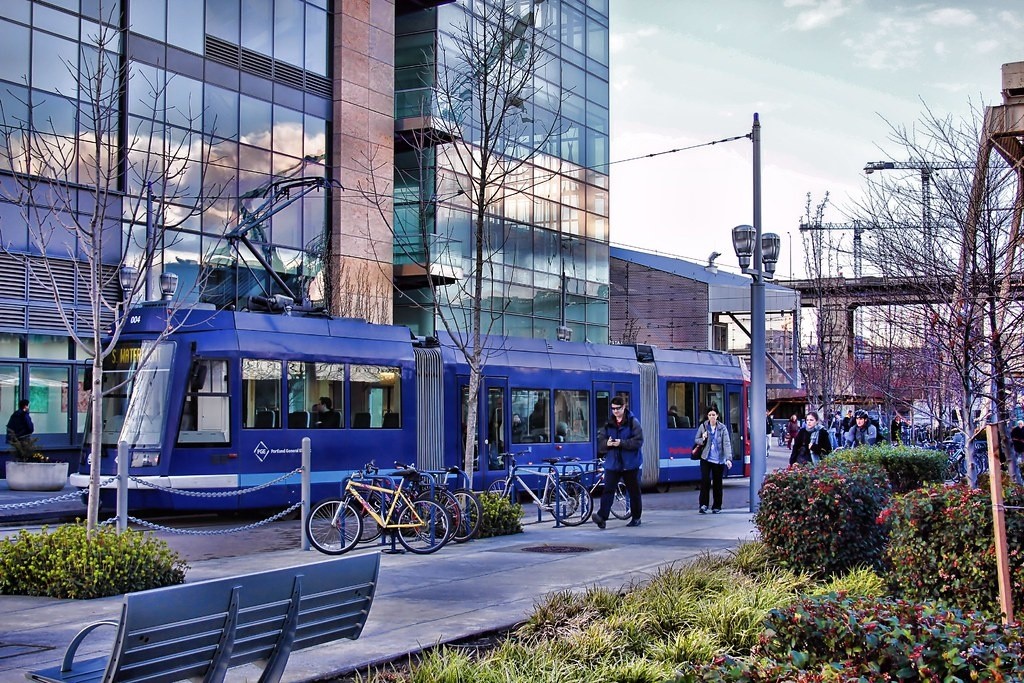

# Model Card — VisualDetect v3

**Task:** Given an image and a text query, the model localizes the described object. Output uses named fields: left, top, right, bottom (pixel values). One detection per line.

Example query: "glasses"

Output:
left=611, top=405, right=623, bottom=410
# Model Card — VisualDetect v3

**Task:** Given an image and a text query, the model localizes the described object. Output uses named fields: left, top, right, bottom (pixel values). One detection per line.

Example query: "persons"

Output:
left=488, top=396, right=542, bottom=469
left=668, top=406, right=682, bottom=428
left=593, top=395, right=644, bottom=528
left=764, top=409, right=937, bottom=466
left=5, top=399, right=34, bottom=448
left=312, top=396, right=341, bottom=428
left=1012, top=420, right=1024, bottom=463
left=555, top=421, right=568, bottom=443
left=694, top=406, right=734, bottom=514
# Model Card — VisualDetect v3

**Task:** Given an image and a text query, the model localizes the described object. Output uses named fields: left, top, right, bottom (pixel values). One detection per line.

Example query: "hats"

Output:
left=670, top=406, right=679, bottom=412
left=556, top=422, right=567, bottom=433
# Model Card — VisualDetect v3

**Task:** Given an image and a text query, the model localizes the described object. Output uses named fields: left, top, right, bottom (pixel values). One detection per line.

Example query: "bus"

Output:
left=68, top=293, right=751, bottom=513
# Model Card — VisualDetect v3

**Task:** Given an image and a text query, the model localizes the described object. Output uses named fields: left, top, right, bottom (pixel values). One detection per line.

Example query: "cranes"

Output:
left=799, top=222, right=960, bottom=364
left=865, top=161, right=1014, bottom=348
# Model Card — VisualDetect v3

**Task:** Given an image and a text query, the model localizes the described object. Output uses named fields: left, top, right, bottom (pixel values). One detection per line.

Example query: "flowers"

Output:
left=6, top=428, right=63, bottom=463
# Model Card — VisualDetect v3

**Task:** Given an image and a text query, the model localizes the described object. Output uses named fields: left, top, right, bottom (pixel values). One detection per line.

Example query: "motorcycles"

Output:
left=777, top=424, right=788, bottom=446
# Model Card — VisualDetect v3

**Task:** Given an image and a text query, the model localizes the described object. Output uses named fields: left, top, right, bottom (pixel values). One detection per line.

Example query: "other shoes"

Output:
left=699, top=506, right=707, bottom=514
left=766, top=450, right=770, bottom=458
left=712, top=509, right=721, bottom=513
left=788, top=446, right=791, bottom=450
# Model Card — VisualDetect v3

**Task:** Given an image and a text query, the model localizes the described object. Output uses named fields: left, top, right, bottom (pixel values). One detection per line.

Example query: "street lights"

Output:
left=730, top=224, right=780, bottom=515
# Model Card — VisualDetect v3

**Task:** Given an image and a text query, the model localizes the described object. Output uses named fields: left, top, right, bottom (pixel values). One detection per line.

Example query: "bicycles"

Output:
left=306, top=458, right=481, bottom=554
left=490, top=447, right=594, bottom=526
left=917, top=437, right=989, bottom=483
left=547, top=455, right=633, bottom=519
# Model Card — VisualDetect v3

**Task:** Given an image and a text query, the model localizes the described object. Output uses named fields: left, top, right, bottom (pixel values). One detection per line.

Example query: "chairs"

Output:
left=668, top=416, right=692, bottom=428
left=288, top=409, right=342, bottom=429
left=255, top=406, right=279, bottom=429
left=382, top=412, right=399, bottom=428
left=352, top=412, right=371, bottom=429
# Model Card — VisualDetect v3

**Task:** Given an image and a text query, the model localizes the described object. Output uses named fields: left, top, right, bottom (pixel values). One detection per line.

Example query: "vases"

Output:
left=6, top=462, right=69, bottom=490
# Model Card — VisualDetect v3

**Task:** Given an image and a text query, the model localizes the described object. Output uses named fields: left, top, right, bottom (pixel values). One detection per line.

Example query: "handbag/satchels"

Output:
left=796, top=428, right=808, bottom=463
left=691, top=422, right=707, bottom=460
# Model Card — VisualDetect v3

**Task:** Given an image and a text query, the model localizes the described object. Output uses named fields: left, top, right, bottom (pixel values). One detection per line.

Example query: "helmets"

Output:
left=855, top=410, right=868, bottom=419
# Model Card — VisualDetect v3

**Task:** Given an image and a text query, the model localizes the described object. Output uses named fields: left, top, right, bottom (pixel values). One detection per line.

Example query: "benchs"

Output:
left=24, top=550, right=383, bottom=683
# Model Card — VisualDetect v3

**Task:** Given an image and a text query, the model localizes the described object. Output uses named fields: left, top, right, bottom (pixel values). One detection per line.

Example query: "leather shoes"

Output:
left=592, top=513, right=606, bottom=529
left=626, top=517, right=641, bottom=527
